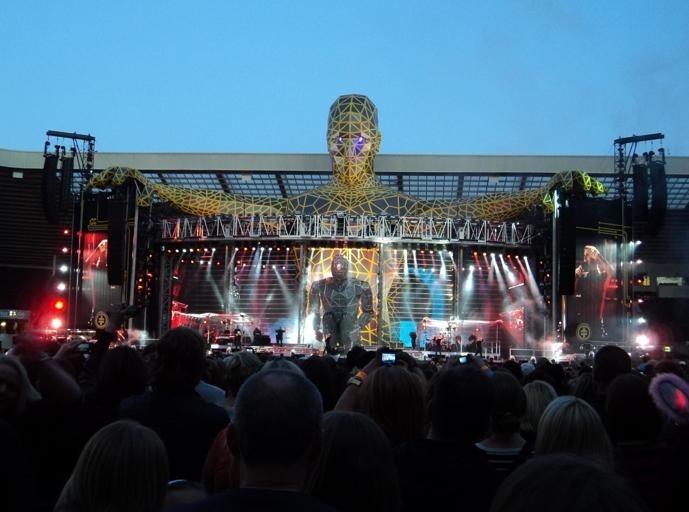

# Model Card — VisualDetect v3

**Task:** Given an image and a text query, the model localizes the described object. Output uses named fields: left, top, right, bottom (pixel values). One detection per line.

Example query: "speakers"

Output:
left=630, top=163, right=667, bottom=241
left=42, top=155, right=59, bottom=225
left=106, top=200, right=125, bottom=283
left=558, top=207, right=577, bottom=296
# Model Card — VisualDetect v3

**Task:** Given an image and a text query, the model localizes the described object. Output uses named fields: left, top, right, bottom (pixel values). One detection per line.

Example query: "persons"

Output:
left=0, top=312, right=688, bottom=512
left=573, top=241, right=615, bottom=337
left=305, top=254, right=374, bottom=351
left=85, top=92, right=606, bottom=222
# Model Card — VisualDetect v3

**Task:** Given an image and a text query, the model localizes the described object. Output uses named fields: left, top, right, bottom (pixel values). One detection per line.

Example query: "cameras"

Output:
left=458, top=356, right=469, bottom=365
left=381, top=349, right=399, bottom=365
